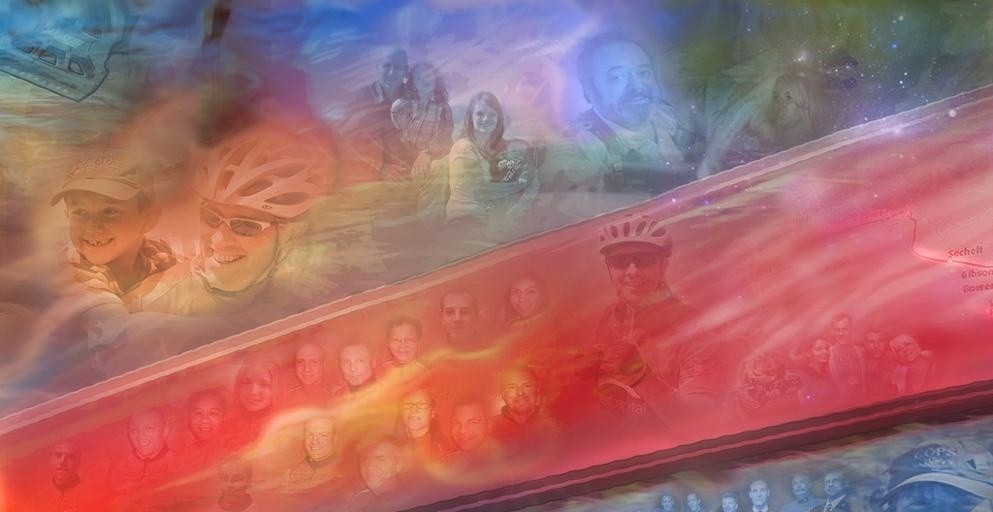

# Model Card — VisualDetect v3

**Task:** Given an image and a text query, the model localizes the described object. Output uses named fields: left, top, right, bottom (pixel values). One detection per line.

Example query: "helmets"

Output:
left=207, top=137, right=323, bottom=219
left=601, top=211, right=672, bottom=256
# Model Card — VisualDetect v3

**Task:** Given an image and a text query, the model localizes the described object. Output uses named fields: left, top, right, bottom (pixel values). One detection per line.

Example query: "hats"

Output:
left=48, top=138, right=156, bottom=210
left=879, top=440, right=992, bottom=502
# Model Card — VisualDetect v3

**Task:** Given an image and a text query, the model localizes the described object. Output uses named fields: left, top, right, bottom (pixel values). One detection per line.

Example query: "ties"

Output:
left=826, top=503, right=832, bottom=511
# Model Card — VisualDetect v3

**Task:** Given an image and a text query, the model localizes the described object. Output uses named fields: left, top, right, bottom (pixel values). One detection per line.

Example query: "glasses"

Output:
left=600, top=250, right=664, bottom=268
left=199, top=199, right=284, bottom=237
left=401, top=398, right=432, bottom=409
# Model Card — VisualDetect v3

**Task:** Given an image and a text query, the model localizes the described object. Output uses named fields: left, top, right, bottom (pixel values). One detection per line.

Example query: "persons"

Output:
left=41, top=215, right=993, bottom=512
left=327, top=42, right=547, bottom=261
left=24, top=149, right=243, bottom=354
left=557, top=33, right=708, bottom=190
left=769, top=75, right=824, bottom=142
left=128, top=124, right=327, bottom=344
